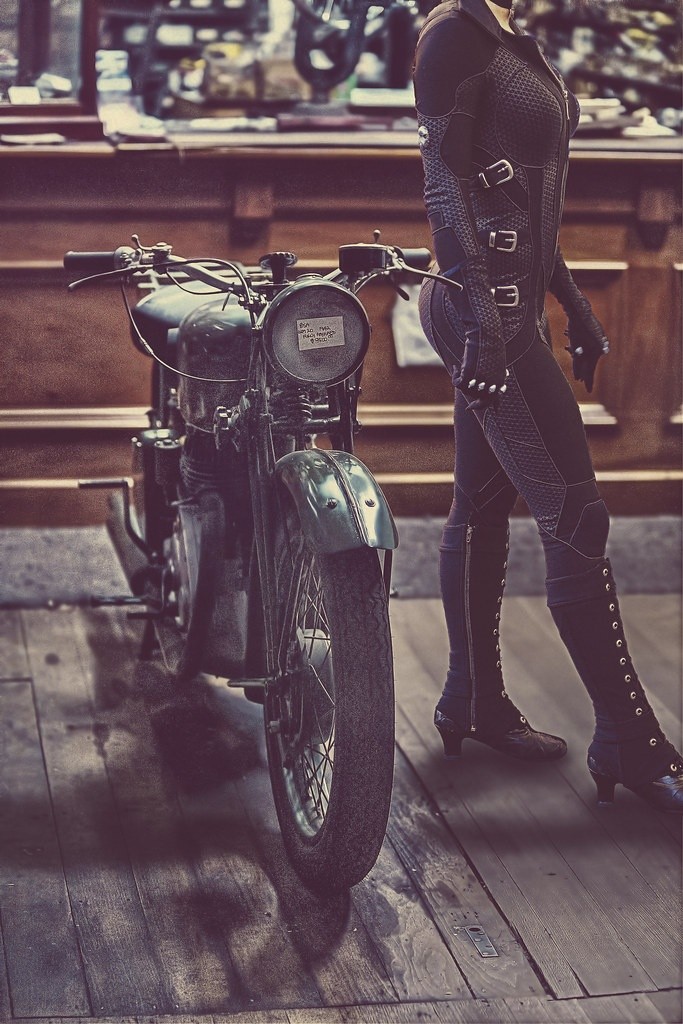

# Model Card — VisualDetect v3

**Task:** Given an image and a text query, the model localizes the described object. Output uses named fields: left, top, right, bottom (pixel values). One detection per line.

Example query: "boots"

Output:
left=544, top=557, right=683, bottom=813
left=432, top=526, right=569, bottom=759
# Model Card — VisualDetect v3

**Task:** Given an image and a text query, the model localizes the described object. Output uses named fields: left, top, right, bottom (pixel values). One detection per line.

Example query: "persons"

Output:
left=413, top=1, right=683, bottom=826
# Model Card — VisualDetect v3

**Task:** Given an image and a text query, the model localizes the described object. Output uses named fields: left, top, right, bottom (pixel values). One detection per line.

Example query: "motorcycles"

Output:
left=61, top=226, right=469, bottom=895
